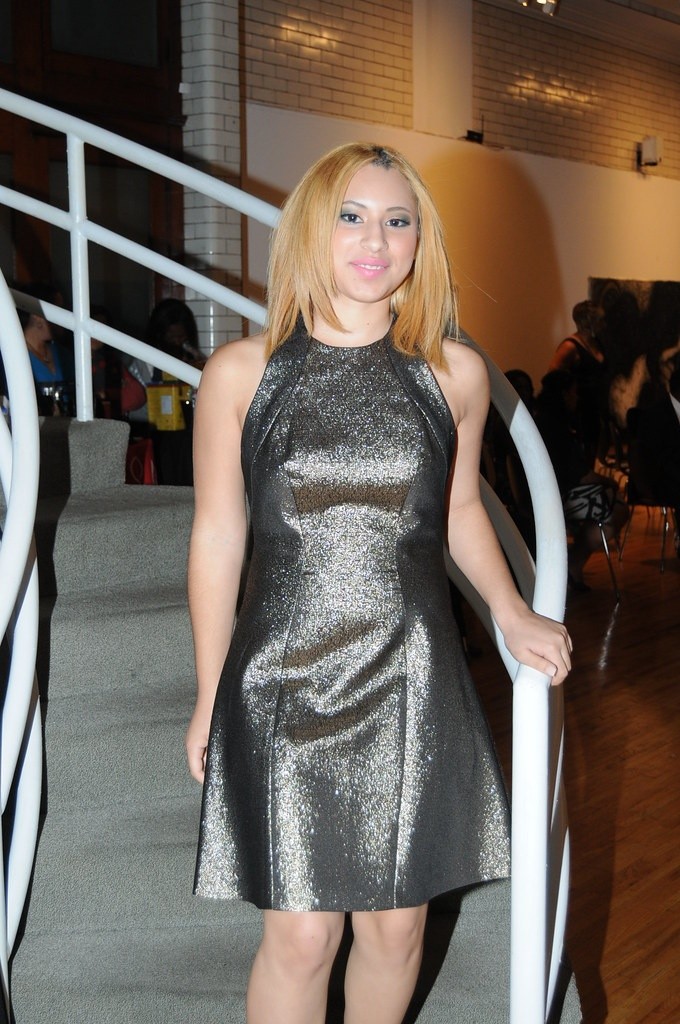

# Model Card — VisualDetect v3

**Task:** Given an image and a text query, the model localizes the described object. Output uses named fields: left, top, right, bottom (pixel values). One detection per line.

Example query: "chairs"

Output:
left=484, top=368, right=680, bottom=601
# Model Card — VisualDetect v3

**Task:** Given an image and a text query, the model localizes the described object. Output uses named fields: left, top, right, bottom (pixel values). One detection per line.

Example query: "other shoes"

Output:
left=568, top=568, right=588, bottom=591
left=465, top=647, right=473, bottom=662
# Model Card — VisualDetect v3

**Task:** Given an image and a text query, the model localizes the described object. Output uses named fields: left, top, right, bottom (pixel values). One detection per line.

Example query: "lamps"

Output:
left=637, top=132, right=662, bottom=169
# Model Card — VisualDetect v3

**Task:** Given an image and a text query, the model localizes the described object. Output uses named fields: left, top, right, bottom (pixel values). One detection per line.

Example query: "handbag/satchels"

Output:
left=114, top=356, right=147, bottom=412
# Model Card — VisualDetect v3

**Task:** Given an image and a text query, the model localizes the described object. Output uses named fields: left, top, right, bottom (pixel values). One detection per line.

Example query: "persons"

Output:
left=24, top=290, right=680, bottom=597
left=184, top=141, right=573, bottom=1024
